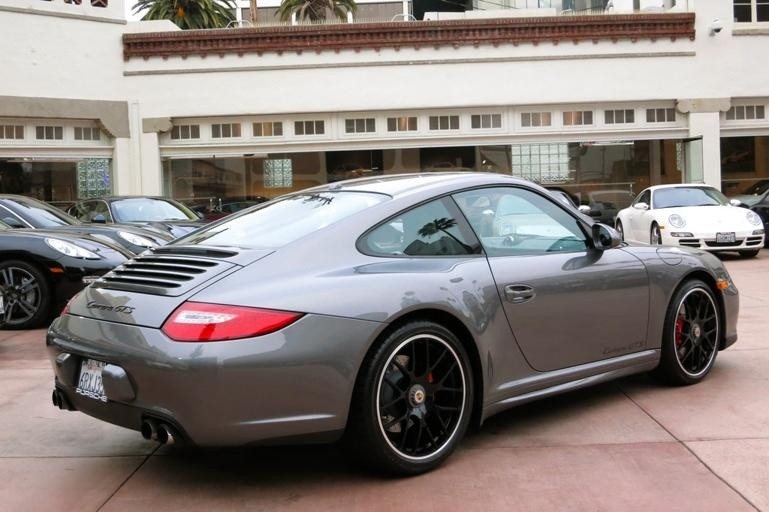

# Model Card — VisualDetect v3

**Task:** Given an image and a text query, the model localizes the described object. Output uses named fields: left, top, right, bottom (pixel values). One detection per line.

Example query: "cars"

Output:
left=46, top=169, right=738, bottom=477
left=485, top=190, right=591, bottom=241
left=730, top=178, right=769, bottom=249
left=613, top=182, right=765, bottom=258
left=1, top=193, right=269, bottom=334
